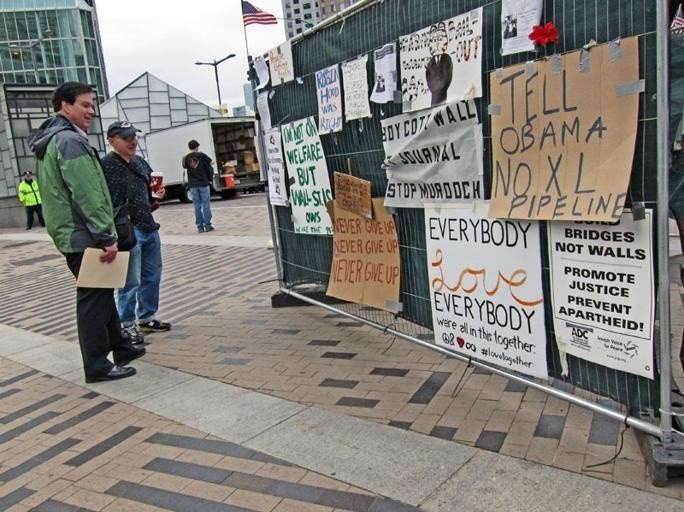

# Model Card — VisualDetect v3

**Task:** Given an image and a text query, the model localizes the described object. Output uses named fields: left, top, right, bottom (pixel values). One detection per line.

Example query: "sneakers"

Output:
left=26, top=226, right=32, bottom=230
left=120, top=321, right=143, bottom=345
left=198, top=226, right=215, bottom=232
left=137, top=319, right=171, bottom=332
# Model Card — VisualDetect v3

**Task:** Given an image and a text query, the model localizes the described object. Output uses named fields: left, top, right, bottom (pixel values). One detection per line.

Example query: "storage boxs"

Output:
left=220, top=150, right=260, bottom=176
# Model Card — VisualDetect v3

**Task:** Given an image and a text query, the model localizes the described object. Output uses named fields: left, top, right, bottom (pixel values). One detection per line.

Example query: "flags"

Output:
left=241, top=0, right=278, bottom=26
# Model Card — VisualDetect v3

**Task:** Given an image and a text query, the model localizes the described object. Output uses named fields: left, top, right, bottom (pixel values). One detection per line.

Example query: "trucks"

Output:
left=142, top=115, right=268, bottom=207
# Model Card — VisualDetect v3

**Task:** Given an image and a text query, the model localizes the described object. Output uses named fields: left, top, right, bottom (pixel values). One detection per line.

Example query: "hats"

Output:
left=108, top=122, right=141, bottom=137
left=24, top=172, right=32, bottom=176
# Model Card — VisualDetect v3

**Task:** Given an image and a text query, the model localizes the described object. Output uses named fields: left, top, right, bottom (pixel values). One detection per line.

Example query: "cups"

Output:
left=150, top=171, right=165, bottom=200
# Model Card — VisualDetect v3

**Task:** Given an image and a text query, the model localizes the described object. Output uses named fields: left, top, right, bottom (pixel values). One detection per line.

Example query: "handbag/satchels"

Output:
left=113, top=205, right=136, bottom=251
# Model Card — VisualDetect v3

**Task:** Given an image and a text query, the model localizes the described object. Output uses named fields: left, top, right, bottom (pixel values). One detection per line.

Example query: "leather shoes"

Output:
left=115, top=348, right=145, bottom=366
left=94, top=366, right=135, bottom=380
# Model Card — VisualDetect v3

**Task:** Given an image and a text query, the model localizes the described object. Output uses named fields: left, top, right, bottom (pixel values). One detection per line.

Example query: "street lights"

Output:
left=9, top=26, right=55, bottom=82
left=193, top=53, right=237, bottom=107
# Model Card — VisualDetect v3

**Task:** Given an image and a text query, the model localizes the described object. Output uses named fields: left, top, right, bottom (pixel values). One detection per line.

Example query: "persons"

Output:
left=98, top=120, right=172, bottom=345
left=17, top=169, right=45, bottom=231
left=26, top=80, right=147, bottom=384
left=503, top=16, right=516, bottom=38
left=181, top=139, right=217, bottom=232
